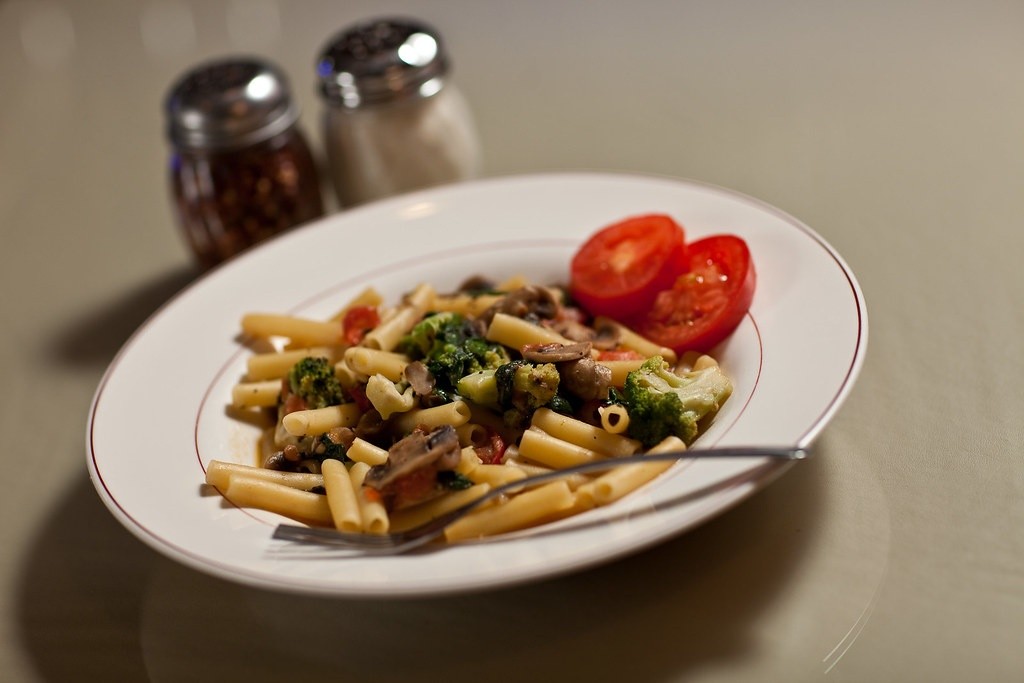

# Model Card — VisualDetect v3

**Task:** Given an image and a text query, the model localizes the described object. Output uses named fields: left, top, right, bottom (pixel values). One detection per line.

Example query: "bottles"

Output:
left=160, top=54, right=327, bottom=273
left=314, top=18, right=481, bottom=209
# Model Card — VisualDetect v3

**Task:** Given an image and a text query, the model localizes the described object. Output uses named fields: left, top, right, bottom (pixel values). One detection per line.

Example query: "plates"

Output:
left=85, top=173, right=869, bottom=599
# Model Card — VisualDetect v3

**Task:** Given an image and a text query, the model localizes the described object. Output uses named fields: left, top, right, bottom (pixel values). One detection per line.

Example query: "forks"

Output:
left=272, top=446, right=812, bottom=553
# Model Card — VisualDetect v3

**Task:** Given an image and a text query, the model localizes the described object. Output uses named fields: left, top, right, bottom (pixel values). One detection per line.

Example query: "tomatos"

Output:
left=569, top=212, right=753, bottom=350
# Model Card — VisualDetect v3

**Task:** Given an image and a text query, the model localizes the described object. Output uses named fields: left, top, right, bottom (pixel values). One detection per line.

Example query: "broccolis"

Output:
left=291, top=311, right=569, bottom=427
left=609, top=356, right=731, bottom=441
